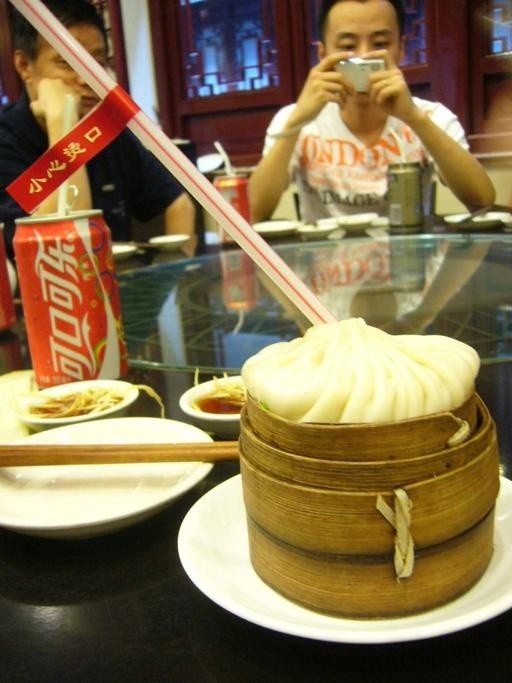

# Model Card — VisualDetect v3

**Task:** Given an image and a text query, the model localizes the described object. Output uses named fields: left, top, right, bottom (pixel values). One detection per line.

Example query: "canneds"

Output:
left=11, top=209, right=130, bottom=390
left=384, top=161, right=425, bottom=234
left=213, top=174, right=251, bottom=247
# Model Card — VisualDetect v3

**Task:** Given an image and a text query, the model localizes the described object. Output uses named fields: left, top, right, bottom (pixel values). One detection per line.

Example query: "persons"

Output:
left=0, top=0, right=195, bottom=266
left=247, top=0, right=495, bottom=225
left=255, top=235, right=491, bottom=336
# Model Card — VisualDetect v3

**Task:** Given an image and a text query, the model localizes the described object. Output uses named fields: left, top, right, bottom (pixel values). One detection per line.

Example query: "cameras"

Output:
left=332, top=58, right=385, bottom=95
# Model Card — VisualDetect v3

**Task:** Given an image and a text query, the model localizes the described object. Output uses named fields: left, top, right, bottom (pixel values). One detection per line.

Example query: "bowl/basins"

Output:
left=111, top=244, right=136, bottom=261
left=248, top=211, right=375, bottom=239
left=178, top=375, right=245, bottom=439
left=149, top=234, right=190, bottom=253
left=15, top=379, right=139, bottom=432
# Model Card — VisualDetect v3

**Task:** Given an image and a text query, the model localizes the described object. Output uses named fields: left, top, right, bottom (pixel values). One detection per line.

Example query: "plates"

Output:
left=175, top=471, right=512, bottom=646
left=0, top=416, right=217, bottom=540
left=443, top=211, right=509, bottom=228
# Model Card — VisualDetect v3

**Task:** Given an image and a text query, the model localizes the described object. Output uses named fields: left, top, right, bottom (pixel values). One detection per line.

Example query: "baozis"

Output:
left=241, top=318, right=480, bottom=426
